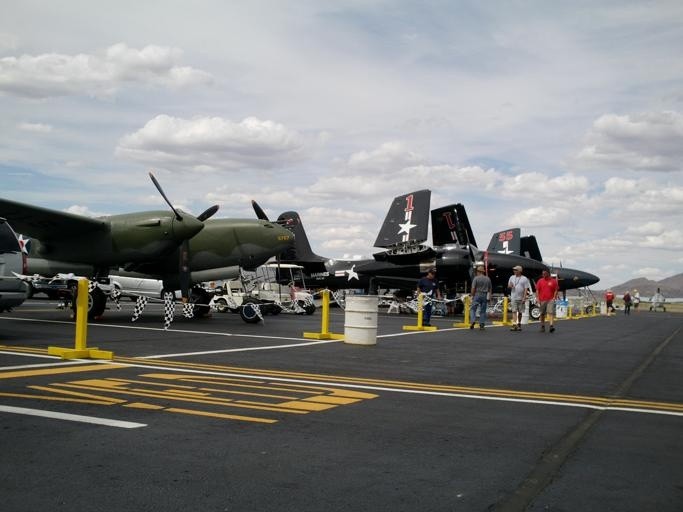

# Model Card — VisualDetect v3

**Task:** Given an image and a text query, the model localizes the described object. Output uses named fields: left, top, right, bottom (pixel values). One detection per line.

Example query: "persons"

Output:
left=507, top=265, right=530, bottom=330
left=623, top=291, right=631, bottom=316
left=469, top=265, right=493, bottom=330
left=605, top=289, right=615, bottom=313
left=417, top=268, right=442, bottom=326
left=632, top=289, right=640, bottom=312
left=536, top=268, right=559, bottom=333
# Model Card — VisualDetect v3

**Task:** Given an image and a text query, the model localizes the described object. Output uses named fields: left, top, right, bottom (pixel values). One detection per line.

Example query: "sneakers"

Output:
left=470, top=321, right=476, bottom=330
left=480, top=325, right=485, bottom=331
left=549, top=326, right=556, bottom=333
left=510, top=323, right=522, bottom=332
left=538, top=326, right=545, bottom=332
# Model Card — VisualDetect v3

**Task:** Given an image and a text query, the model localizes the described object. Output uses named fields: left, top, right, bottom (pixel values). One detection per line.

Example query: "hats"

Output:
left=428, top=269, right=436, bottom=273
left=512, top=265, right=522, bottom=272
left=476, top=266, right=485, bottom=272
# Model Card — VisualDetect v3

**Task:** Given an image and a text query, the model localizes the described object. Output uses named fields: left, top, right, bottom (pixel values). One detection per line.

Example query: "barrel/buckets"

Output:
left=556, top=301, right=568, bottom=318
left=343, top=297, right=379, bottom=345
left=601, top=301, right=607, bottom=315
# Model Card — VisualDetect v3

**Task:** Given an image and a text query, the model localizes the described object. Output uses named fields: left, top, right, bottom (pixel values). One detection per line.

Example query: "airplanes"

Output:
left=616, top=288, right=683, bottom=312
